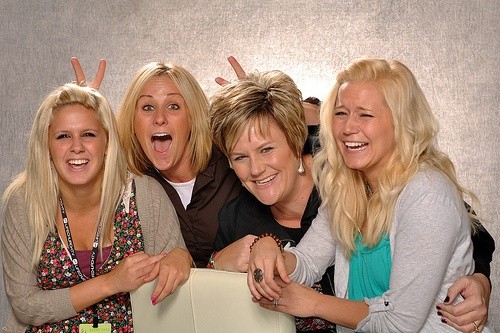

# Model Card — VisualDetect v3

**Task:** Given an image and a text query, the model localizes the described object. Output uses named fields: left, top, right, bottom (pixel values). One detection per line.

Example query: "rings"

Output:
left=273, top=299, right=278, bottom=308
left=252, top=268, right=264, bottom=282
left=473, top=321, right=480, bottom=332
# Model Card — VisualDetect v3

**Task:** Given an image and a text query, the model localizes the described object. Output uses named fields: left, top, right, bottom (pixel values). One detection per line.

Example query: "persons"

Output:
left=71, top=56, right=320, bottom=269
left=0, top=83, right=197, bottom=333
left=245, top=58, right=495, bottom=333
left=207, top=71, right=495, bottom=333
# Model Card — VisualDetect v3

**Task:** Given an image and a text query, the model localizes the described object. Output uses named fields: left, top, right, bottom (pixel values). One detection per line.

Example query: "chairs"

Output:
left=130, top=269, right=296, bottom=333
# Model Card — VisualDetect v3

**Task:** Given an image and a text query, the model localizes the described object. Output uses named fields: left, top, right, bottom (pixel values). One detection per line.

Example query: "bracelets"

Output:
left=249, top=233, right=285, bottom=254
left=208, top=252, right=218, bottom=269
